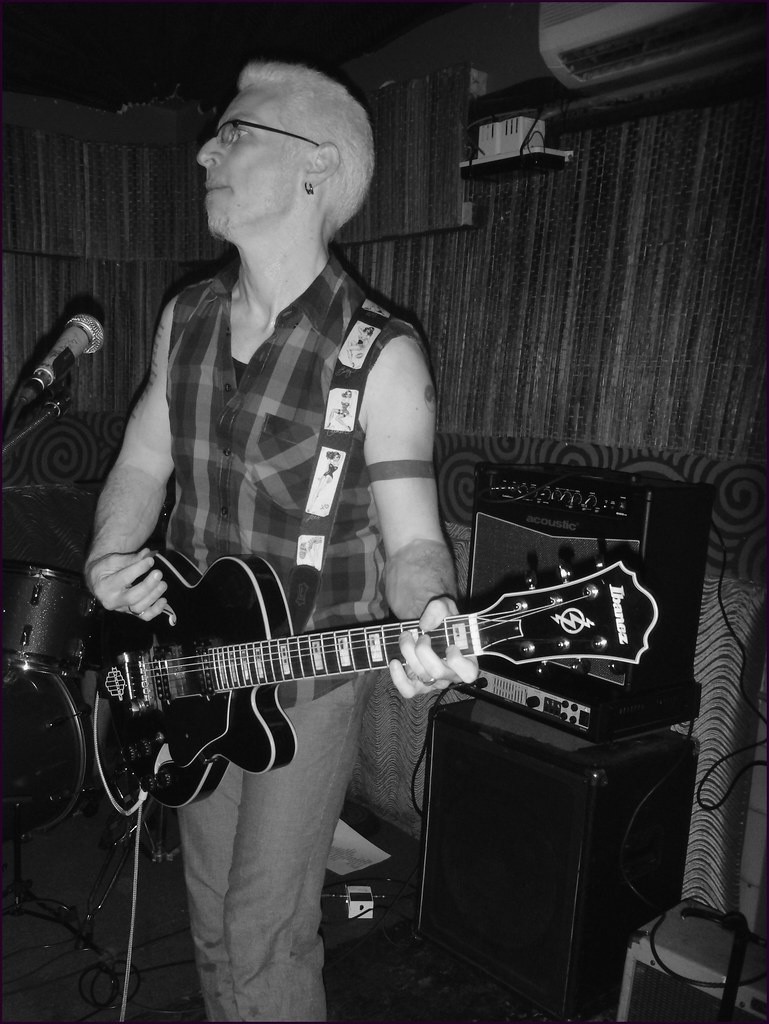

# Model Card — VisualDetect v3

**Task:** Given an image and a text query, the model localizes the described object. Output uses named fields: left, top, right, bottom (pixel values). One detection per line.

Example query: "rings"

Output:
left=418, top=677, right=439, bottom=687
left=127, top=604, right=145, bottom=617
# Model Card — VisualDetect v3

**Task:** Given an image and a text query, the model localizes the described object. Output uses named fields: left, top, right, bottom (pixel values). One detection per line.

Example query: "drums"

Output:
left=0, top=667, right=99, bottom=839
left=0, top=556, right=103, bottom=681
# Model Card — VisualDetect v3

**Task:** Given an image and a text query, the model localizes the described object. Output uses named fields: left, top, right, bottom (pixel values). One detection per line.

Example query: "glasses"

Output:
left=214, top=119, right=321, bottom=151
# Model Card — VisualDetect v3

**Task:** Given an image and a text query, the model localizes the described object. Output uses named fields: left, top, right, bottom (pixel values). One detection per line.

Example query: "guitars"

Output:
left=96, top=547, right=661, bottom=815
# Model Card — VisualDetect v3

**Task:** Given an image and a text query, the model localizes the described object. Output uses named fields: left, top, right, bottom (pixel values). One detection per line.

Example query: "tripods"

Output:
left=1, top=766, right=165, bottom=953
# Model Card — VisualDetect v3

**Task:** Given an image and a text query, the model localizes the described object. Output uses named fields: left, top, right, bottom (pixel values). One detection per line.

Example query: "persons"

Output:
left=83, top=63, right=481, bottom=1022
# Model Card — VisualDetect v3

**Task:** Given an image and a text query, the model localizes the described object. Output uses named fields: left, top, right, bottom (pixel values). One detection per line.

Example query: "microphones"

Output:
left=20, top=314, right=105, bottom=405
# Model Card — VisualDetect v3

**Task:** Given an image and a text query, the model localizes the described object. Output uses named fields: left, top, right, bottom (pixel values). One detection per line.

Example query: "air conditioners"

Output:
left=537, top=2, right=766, bottom=91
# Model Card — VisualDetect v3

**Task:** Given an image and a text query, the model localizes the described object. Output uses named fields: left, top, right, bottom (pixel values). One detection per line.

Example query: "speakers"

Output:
left=466, top=461, right=716, bottom=744
left=616, top=896, right=767, bottom=1024
left=416, top=698, right=696, bottom=1024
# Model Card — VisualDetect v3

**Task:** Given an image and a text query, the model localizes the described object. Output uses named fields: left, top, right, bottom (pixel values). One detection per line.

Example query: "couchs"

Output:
left=2, top=412, right=765, bottom=843
